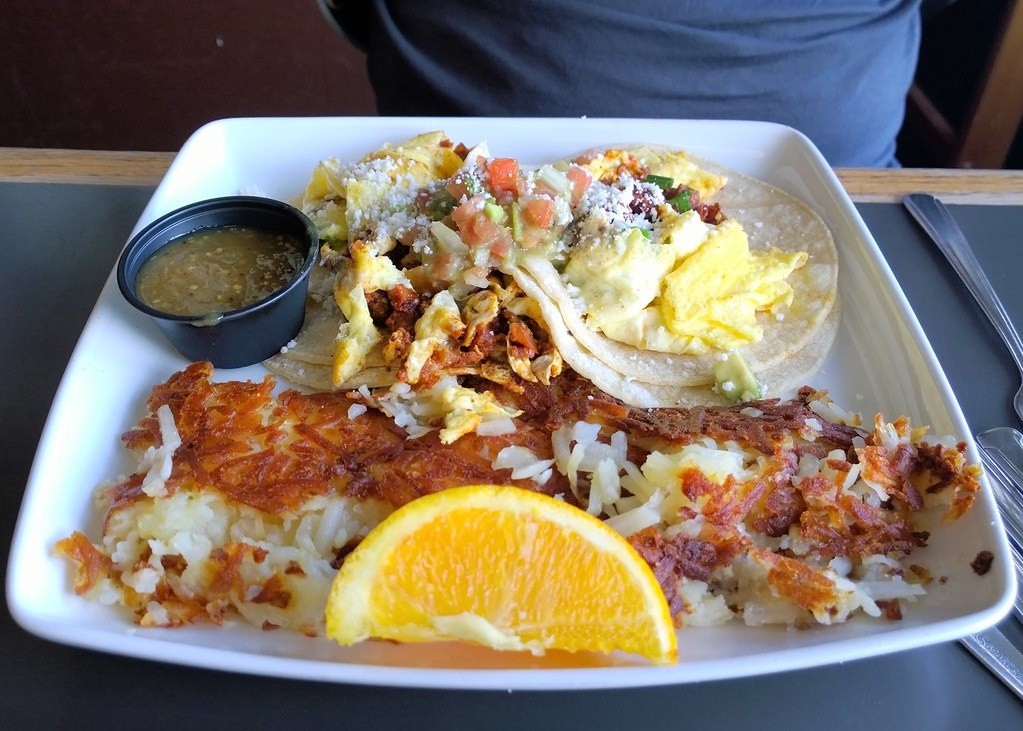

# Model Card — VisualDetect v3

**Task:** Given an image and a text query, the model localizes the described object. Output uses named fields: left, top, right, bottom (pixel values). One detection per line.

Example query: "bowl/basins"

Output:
left=116, top=196, right=319, bottom=369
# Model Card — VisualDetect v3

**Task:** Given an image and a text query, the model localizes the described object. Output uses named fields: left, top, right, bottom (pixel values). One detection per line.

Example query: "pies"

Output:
left=266, top=145, right=840, bottom=403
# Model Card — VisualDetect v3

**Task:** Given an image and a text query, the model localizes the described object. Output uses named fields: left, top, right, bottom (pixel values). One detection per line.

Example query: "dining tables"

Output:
left=0, top=148, right=1023, bottom=731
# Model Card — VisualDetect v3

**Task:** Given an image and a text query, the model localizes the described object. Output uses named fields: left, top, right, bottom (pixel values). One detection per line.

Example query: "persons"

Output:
left=320, top=0, right=923, bottom=169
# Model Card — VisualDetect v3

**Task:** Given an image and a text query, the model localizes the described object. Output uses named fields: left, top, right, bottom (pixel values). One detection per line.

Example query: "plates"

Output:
left=5, top=116, right=1017, bottom=691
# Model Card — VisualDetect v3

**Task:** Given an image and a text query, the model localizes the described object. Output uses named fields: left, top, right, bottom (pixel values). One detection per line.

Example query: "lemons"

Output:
left=326, top=484, right=677, bottom=662
